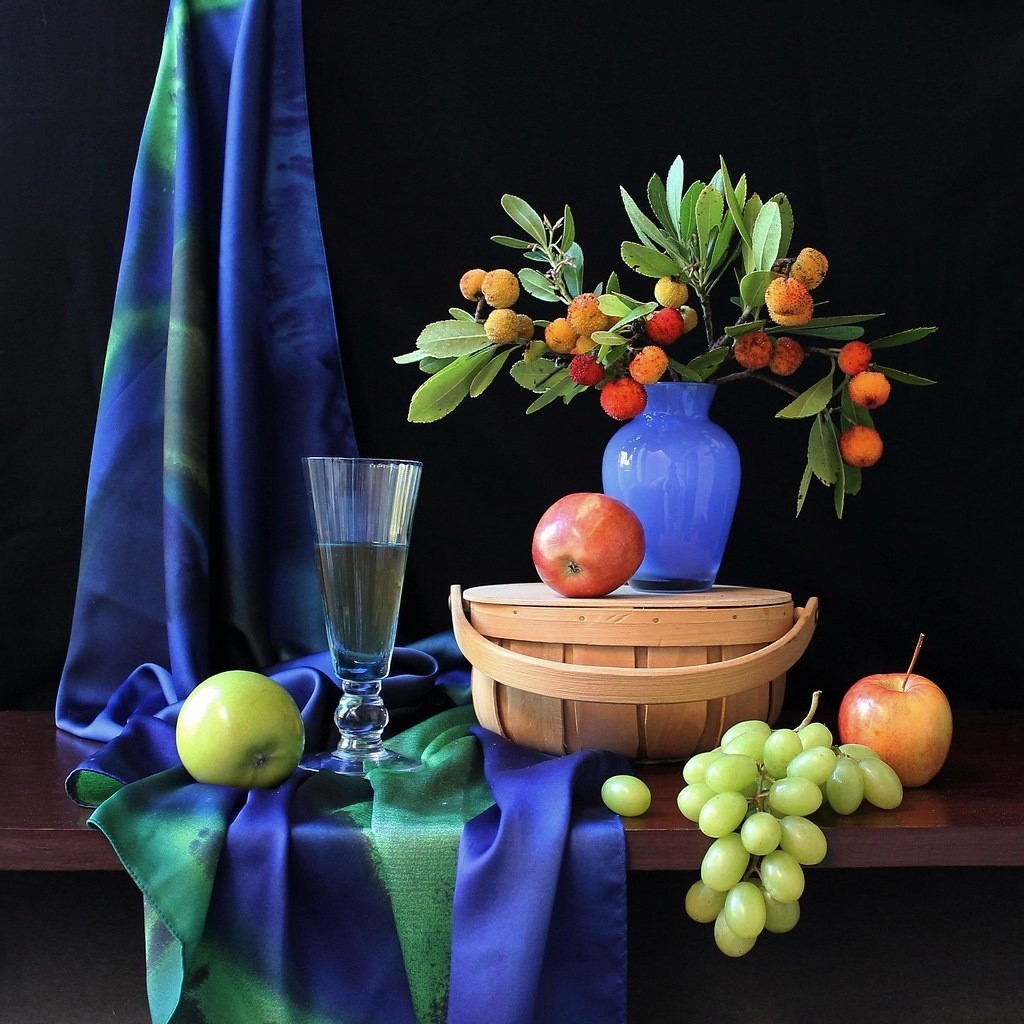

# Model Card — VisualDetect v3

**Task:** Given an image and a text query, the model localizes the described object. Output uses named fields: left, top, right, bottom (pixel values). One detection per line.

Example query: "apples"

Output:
left=837, top=633, right=952, bottom=787
left=531, top=493, right=646, bottom=597
left=175, top=670, right=305, bottom=792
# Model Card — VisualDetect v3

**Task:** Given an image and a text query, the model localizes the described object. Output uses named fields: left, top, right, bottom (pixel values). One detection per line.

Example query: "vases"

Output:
left=601, top=381, right=742, bottom=593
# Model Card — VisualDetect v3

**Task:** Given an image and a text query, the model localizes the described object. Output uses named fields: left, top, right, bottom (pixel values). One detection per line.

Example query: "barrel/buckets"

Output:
left=447, top=582, right=819, bottom=765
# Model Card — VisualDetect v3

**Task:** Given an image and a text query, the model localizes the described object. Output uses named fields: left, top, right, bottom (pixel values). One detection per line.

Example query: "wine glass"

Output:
left=299, top=456, right=428, bottom=777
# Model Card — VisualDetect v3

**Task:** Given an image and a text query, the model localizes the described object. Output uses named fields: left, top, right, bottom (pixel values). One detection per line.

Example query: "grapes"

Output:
left=600, top=775, right=652, bottom=817
left=678, top=719, right=904, bottom=960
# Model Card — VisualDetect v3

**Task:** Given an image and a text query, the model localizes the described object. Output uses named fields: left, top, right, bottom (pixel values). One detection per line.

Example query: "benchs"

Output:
left=0, top=702, right=1024, bottom=868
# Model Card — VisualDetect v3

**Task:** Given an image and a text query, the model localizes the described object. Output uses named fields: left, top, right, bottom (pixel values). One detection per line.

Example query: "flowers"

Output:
left=394, top=151, right=939, bottom=521
